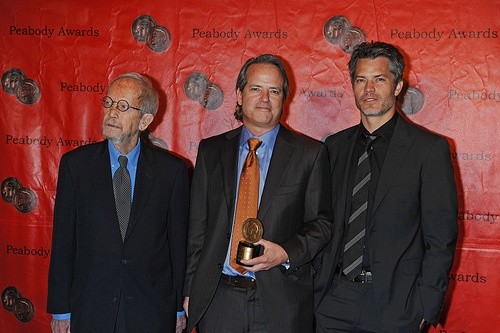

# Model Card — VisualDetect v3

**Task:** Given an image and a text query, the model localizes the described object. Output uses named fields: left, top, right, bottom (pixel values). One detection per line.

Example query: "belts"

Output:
left=221, top=273, right=256, bottom=289
left=335, top=265, right=371, bottom=283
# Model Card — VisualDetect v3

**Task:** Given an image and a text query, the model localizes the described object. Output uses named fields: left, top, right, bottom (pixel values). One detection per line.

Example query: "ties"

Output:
left=112, top=155, right=131, bottom=243
left=342, top=134, right=376, bottom=281
left=229, top=137, right=263, bottom=274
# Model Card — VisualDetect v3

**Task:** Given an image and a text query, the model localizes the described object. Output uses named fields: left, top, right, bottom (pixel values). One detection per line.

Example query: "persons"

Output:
left=183, top=55, right=334, bottom=333
left=45, top=72, right=191, bottom=333
left=312, top=41, right=459, bottom=333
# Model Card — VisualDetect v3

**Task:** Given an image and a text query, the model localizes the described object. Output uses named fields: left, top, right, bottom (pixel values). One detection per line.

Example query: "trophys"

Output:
left=236, top=218, right=264, bottom=267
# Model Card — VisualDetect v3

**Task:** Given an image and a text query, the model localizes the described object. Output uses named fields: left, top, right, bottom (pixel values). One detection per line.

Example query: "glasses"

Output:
left=100, top=96, right=152, bottom=114
